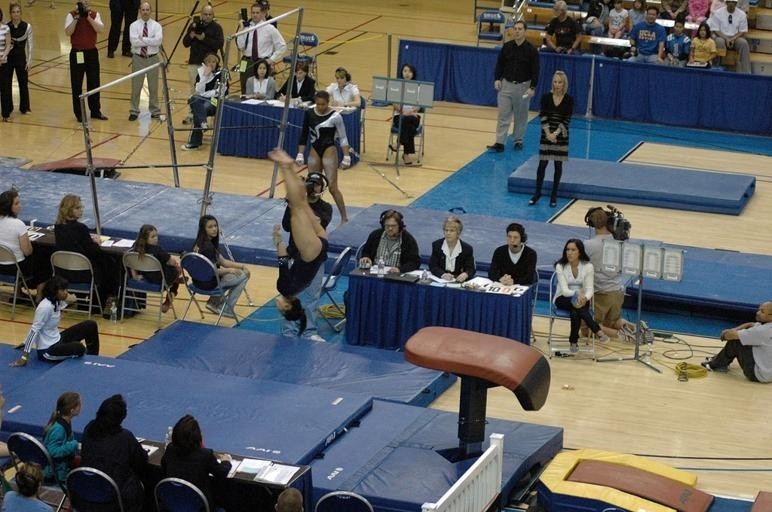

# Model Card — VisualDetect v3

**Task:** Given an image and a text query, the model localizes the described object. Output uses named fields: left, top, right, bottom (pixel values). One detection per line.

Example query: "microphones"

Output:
left=394, top=231, right=400, bottom=236
left=514, top=243, right=523, bottom=247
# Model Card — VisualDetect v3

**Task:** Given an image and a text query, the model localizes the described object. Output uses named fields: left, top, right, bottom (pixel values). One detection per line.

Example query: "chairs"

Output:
left=155, top=479, right=210, bottom=511
left=547, top=271, right=596, bottom=361
left=189, top=78, right=230, bottom=146
left=121, top=253, right=178, bottom=331
left=67, top=468, right=147, bottom=511
left=287, top=28, right=320, bottom=85
left=50, top=250, right=105, bottom=321
left=1, top=245, right=37, bottom=320
left=315, top=491, right=373, bottom=512
left=7, top=433, right=70, bottom=512
left=345, top=242, right=539, bottom=348
left=385, top=99, right=429, bottom=170
left=305, top=246, right=352, bottom=333
left=357, top=94, right=365, bottom=138
left=180, top=252, right=240, bottom=327
left=477, top=10, right=506, bottom=47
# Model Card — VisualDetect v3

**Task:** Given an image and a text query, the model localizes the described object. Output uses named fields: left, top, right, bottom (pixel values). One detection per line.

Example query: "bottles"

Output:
left=377, top=256, right=385, bottom=278
left=644, top=342, right=652, bottom=363
left=109, top=301, right=118, bottom=324
left=420, top=268, right=428, bottom=282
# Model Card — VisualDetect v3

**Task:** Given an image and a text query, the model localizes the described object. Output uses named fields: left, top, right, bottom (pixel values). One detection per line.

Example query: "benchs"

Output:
left=507, top=0, right=772, bottom=75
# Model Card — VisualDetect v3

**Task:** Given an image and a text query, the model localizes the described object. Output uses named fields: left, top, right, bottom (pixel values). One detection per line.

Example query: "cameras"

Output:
left=191, top=16, right=205, bottom=36
left=77, top=1, right=91, bottom=17
left=240, top=8, right=250, bottom=27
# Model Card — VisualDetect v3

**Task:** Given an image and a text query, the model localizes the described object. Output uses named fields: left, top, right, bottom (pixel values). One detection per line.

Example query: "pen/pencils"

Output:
left=260, top=461, right=274, bottom=479
left=32, top=234, right=39, bottom=239
left=281, top=93, right=284, bottom=96
left=400, top=273, right=405, bottom=277
left=28, top=234, right=35, bottom=238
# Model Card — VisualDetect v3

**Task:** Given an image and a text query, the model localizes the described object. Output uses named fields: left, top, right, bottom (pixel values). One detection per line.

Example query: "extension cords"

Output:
left=653, top=330, right=672, bottom=338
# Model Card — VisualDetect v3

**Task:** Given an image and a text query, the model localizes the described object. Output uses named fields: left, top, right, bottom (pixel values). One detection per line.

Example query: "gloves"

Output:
left=295, top=154, right=304, bottom=166
left=340, top=156, right=351, bottom=169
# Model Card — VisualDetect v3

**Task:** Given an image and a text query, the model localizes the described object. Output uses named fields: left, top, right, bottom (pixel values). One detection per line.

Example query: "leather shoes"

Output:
left=486, top=143, right=504, bottom=152
left=129, top=115, right=136, bottom=121
left=151, top=114, right=160, bottom=119
left=91, top=112, right=108, bottom=120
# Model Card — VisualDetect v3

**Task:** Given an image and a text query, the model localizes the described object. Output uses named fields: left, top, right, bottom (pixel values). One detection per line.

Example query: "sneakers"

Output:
left=570, top=342, right=579, bottom=354
left=181, top=144, right=199, bottom=150
left=701, top=356, right=729, bottom=372
left=597, top=333, right=610, bottom=344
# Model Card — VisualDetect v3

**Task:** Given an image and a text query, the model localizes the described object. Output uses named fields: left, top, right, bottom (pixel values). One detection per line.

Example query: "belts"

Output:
left=138, top=54, right=155, bottom=58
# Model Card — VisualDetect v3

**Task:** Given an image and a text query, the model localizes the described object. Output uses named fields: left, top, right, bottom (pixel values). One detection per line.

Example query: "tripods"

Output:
left=596, top=275, right=663, bottom=373
left=368, top=103, right=422, bottom=177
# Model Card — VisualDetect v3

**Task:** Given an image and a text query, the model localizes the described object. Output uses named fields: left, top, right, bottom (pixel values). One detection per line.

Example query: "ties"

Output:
left=140, top=23, right=148, bottom=56
left=252, top=30, right=258, bottom=61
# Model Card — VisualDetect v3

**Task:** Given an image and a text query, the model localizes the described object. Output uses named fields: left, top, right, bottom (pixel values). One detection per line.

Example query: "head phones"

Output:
left=584, top=207, right=602, bottom=228
left=510, top=225, right=527, bottom=242
left=379, top=209, right=404, bottom=231
left=204, top=51, right=221, bottom=69
left=308, top=172, right=329, bottom=196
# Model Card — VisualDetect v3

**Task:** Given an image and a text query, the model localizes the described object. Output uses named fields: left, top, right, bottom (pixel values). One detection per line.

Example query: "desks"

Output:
left=24, top=226, right=146, bottom=318
left=76, top=421, right=313, bottom=511
left=213, top=92, right=364, bottom=169
